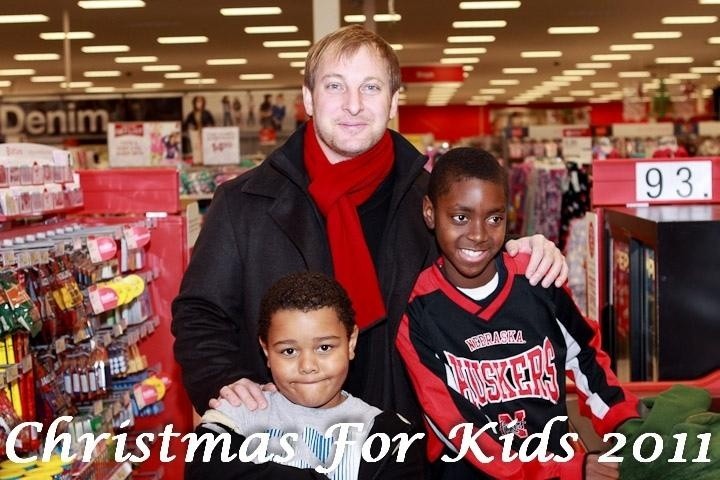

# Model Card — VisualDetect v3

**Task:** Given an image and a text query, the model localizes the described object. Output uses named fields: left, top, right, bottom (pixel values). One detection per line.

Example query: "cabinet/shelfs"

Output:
left=586, top=207, right=719, bottom=382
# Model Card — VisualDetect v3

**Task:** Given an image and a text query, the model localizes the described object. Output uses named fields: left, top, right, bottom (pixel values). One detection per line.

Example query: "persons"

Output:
left=184, top=271, right=427, bottom=479
left=171, top=25, right=569, bottom=419
left=181, top=92, right=287, bottom=134
left=395, top=147, right=641, bottom=480
